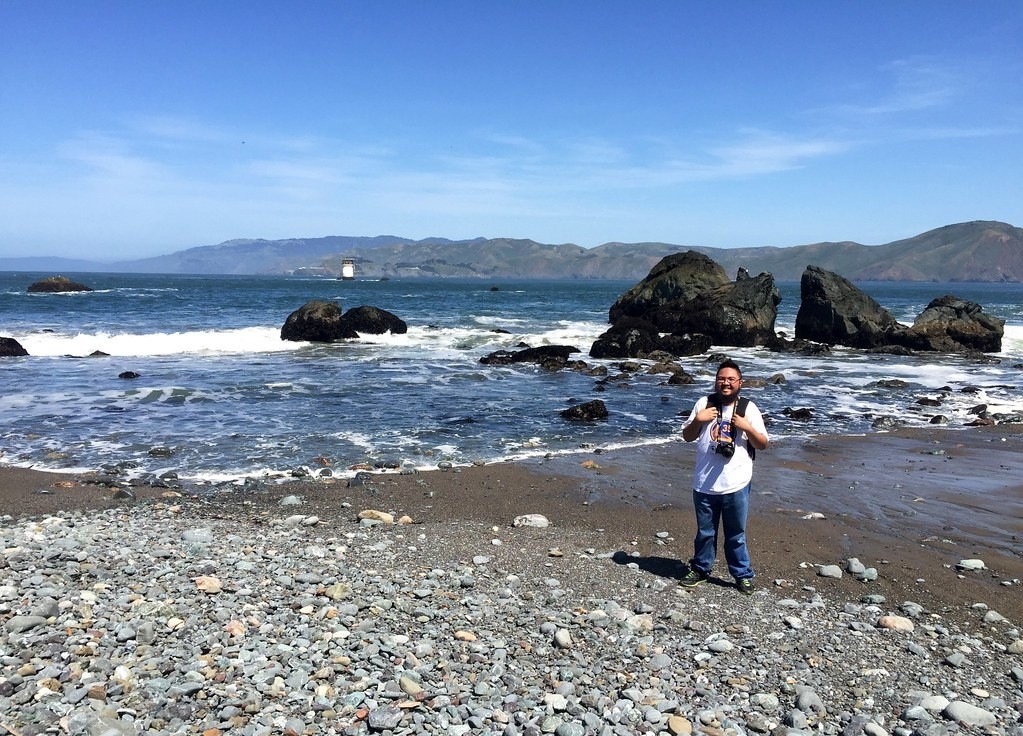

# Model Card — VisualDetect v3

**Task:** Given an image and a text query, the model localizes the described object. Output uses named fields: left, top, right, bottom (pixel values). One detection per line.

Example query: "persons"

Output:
left=678, top=358, right=769, bottom=595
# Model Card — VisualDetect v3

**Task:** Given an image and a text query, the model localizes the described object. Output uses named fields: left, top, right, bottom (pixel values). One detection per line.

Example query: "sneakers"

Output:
left=681, top=570, right=707, bottom=587
left=736, top=577, right=754, bottom=595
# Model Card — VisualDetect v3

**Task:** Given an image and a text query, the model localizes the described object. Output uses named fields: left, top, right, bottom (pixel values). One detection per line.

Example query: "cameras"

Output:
left=715, top=441, right=735, bottom=458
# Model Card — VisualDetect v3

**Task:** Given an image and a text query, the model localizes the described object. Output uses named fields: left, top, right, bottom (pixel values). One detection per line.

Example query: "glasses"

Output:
left=716, top=376, right=740, bottom=384
